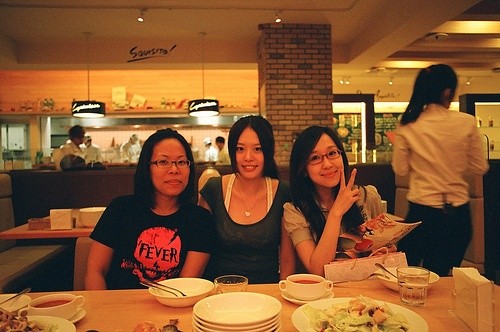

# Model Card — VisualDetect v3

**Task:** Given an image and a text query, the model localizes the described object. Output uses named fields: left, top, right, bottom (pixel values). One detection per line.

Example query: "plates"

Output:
left=23, top=315, right=77, bottom=332
left=69, top=308, right=85, bottom=324
left=374, top=267, right=440, bottom=293
left=291, top=297, right=429, bottom=332
left=281, top=290, right=334, bottom=305
left=192, top=293, right=282, bottom=332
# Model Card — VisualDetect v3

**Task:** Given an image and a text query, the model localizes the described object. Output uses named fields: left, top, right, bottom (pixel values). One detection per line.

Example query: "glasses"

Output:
left=308, top=149, right=342, bottom=165
left=149, top=159, right=190, bottom=169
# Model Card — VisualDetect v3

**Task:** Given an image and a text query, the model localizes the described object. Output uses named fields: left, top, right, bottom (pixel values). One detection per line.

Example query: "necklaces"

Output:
left=236, top=180, right=263, bottom=218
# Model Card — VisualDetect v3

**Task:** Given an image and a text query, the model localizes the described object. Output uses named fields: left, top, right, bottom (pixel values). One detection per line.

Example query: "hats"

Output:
left=204, top=138, right=211, bottom=145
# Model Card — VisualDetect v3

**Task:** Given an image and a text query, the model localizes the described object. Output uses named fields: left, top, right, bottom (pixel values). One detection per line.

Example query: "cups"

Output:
left=396, top=267, right=430, bottom=307
left=79, top=207, right=107, bottom=228
left=18, top=294, right=85, bottom=321
left=214, top=275, right=249, bottom=294
left=279, top=273, right=334, bottom=300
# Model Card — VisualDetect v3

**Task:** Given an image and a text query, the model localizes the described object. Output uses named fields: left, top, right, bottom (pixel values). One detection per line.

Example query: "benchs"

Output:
left=0, top=174, right=63, bottom=290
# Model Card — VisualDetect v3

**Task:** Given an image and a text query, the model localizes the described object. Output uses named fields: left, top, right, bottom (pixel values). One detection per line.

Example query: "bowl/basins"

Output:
left=0, top=293, right=32, bottom=317
left=148, top=278, right=215, bottom=308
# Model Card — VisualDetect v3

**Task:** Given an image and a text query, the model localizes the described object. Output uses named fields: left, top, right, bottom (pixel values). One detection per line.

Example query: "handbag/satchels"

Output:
left=324, top=247, right=408, bottom=284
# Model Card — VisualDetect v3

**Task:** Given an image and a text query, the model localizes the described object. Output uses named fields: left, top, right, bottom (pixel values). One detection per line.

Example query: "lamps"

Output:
left=137, top=9, right=144, bottom=22
left=424, top=31, right=448, bottom=42
left=274, top=12, right=281, bottom=22
left=71, top=32, right=105, bottom=118
left=187, top=31, right=220, bottom=118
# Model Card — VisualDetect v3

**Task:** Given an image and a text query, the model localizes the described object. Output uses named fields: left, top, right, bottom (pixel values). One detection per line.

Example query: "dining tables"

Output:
left=24, top=277, right=500, bottom=332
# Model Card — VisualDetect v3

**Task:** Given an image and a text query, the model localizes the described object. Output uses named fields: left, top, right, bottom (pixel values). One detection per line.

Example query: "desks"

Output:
left=0, top=216, right=93, bottom=288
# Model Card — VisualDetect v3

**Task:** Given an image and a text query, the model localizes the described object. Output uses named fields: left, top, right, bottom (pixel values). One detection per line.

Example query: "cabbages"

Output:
left=298, top=296, right=408, bottom=332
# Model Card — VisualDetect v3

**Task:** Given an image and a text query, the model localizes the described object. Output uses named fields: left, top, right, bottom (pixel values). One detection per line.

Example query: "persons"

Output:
left=196, top=114, right=297, bottom=285
left=120, top=133, right=140, bottom=164
left=55, top=124, right=86, bottom=170
left=83, top=127, right=216, bottom=290
left=214, top=135, right=231, bottom=165
left=202, top=138, right=219, bottom=163
left=83, top=135, right=101, bottom=163
left=390, top=61, right=489, bottom=277
left=282, top=122, right=398, bottom=278
